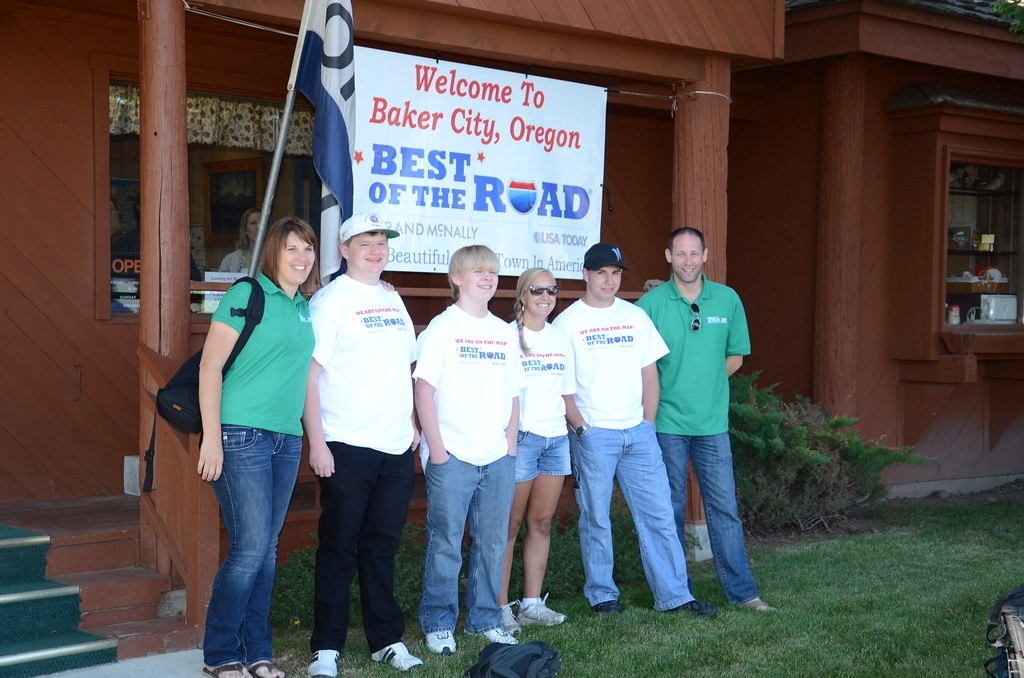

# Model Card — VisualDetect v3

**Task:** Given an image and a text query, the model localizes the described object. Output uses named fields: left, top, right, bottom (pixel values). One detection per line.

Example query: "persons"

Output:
left=300, top=212, right=421, bottom=678
left=110, top=186, right=203, bottom=305
left=197, top=218, right=316, bottom=678
left=551, top=243, right=716, bottom=618
left=218, top=207, right=266, bottom=273
left=634, top=227, right=777, bottom=613
left=500, top=268, right=569, bottom=636
left=413, top=244, right=525, bottom=657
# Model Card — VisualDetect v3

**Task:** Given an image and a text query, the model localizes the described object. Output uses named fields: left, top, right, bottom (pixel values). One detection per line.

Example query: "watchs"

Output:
left=577, top=425, right=592, bottom=438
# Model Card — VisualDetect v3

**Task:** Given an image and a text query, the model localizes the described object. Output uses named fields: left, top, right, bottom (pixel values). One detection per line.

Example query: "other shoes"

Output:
left=743, top=596, right=780, bottom=616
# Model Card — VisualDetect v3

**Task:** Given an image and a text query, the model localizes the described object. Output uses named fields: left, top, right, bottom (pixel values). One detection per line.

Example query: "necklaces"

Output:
left=523, top=322, right=544, bottom=331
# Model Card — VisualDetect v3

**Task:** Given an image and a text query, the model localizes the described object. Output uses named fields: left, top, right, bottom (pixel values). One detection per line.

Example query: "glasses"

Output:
left=527, top=285, right=559, bottom=296
left=690, top=302, right=702, bottom=333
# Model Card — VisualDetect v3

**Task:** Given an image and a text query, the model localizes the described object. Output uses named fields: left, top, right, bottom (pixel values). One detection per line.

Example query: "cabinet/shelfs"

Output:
left=941, top=145, right=1024, bottom=355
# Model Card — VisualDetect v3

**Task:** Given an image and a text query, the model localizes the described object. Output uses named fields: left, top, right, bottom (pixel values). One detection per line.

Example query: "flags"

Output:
left=295, top=0, right=358, bottom=288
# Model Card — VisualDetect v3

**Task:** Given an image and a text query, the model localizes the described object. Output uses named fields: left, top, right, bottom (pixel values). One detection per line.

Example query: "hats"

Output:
left=340, top=213, right=401, bottom=246
left=582, top=242, right=628, bottom=273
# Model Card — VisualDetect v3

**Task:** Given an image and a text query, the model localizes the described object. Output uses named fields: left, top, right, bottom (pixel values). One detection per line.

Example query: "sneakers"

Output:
left=463, top=626, right=519, bottom=646
left=372, top=642, right=424, bottom=672
left=663, top=601, right=718, bottom=621
left=425, top=630, right=458, bottom=655
left=591, top=600, right=623, bottom=616
left=307, top=650, right=340, bottom=677
left=498, top=601, right=523, bottom=636
left=515, top=592, right=568, bottom=626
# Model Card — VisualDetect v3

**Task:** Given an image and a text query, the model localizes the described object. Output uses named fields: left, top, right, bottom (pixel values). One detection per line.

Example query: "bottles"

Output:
left=947, top=304, right=960, bottom=324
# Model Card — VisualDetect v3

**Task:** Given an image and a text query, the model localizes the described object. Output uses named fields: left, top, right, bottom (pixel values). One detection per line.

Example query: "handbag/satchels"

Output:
left=143, top=276, right=265, bottom=491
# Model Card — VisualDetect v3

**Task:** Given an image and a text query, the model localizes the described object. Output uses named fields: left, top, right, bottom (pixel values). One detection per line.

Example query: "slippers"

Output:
left=248, top=663, right=285, bottom=678
left=201, top=661, right=254, bottom=678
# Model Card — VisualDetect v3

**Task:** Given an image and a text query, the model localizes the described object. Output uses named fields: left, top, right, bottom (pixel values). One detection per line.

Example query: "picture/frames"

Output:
left=200, top=157, right=263, bottom=248
